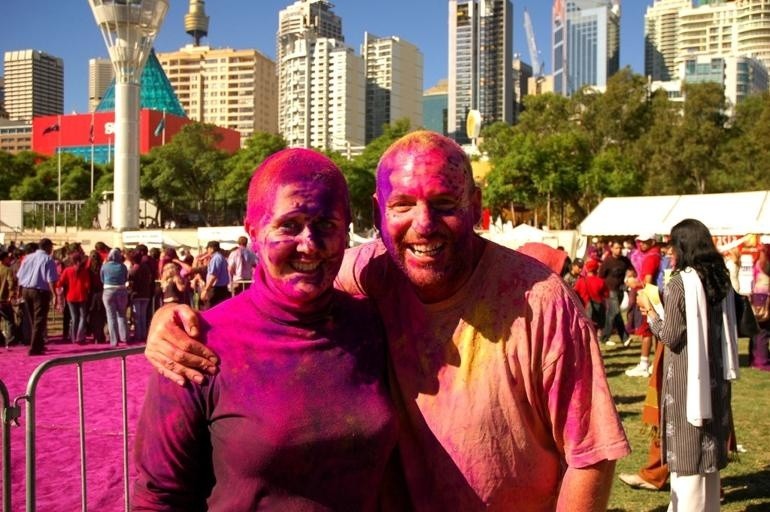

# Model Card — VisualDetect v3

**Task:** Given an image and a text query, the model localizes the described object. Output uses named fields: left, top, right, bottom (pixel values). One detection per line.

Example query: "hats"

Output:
left=516, top=239, right=569, bottom=278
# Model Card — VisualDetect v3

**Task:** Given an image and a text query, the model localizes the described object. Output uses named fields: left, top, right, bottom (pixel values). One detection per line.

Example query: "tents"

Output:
left=577, top=190, right=770, bottom=268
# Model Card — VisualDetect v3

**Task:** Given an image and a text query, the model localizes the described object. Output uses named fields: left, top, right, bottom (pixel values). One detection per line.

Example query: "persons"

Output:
left=1, top=225, right=259, bottom=357
left=145, top=129, right=631, bottom=511
left=635, top=217, right=739, bottom=512
left=616, top=341, right=666, bottom=489
left=512, top=224, right=770, bottom=378
left=131, top=148, right=400, bottom=510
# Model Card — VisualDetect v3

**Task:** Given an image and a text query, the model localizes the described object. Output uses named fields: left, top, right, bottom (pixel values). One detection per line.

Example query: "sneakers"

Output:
left=624, top=363, right=656, bottom=379
left=617, top=472, right=660, bottom=491
left=624, top=336, right=633, bottom=348
left=605, top=340, right=616, bottom=346
